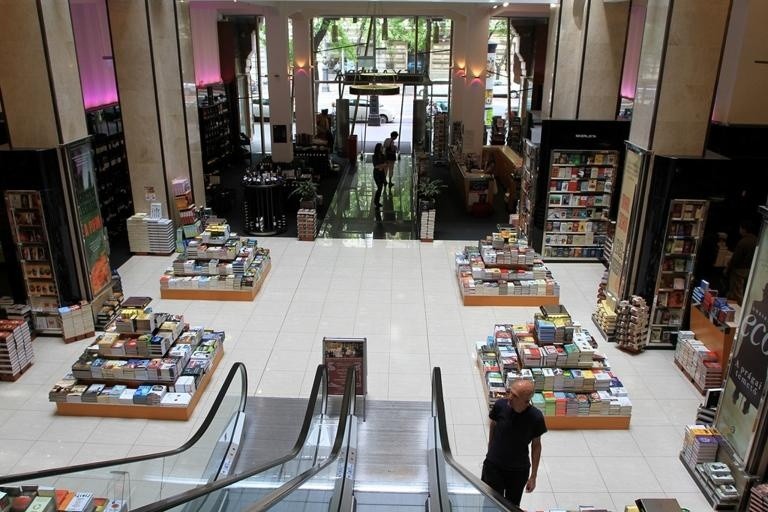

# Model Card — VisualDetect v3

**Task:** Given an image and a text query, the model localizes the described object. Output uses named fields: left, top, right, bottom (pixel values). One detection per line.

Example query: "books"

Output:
left=160, top=218, right=270, bottom=289
left=434, top=111, right=623, bottom=296
left=49, top=293, right=225, bottom=404
left=172, top=177, right=212, bottom=253
left=2, top=296, right=34, bottom=375
left=646, top=198, right=768, bottom=512
left=1, top=485, right=127, bottom=511
left=5, top=190, right=63, bottom=328
left=476, top=303, right=633, bottom=417
left=127, top=202, right=176, bottom=253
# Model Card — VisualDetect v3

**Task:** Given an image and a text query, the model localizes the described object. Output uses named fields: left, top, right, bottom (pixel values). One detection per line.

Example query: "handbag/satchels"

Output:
left=385, top=139, right=396, bottom=162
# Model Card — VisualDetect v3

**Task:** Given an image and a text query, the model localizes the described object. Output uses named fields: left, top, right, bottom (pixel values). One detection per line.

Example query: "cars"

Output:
left=491, top=80, right=522, bottom=99
left=433, top=100, right=449, bottom=113
left=332, top=98, right=396, bottom=124
left=252, top=96, right=270, bottom=121
left=417, top=79, right=451, bottom=96
left=619, top=87, right=658, bottom=119
left=504, top=89, right=532, bottom=116
left=182, top=82, right=198, bottom=96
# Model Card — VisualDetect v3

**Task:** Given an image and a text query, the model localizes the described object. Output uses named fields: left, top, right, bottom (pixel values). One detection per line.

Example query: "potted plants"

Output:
left=292, top=178, right=318, bottom=209
left=414, top=179, right=449, bottom=210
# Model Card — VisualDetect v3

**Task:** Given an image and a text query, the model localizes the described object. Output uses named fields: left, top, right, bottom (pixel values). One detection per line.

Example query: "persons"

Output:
left=481, top=380, right=547, bottom=512
left=317, top=109, right=333, bottom=152
left=372, top=143, right=386, bottom=207
left=382, top=131, right=399, bottom=186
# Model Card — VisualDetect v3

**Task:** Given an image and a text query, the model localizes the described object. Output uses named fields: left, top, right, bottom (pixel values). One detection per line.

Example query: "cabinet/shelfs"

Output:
left=644, top=197, right=709, bottom=349
left=517, top=138, right=625, bottom=264
